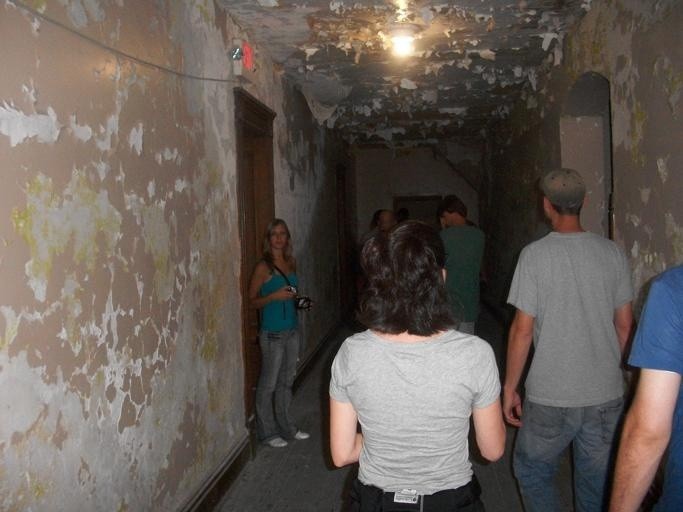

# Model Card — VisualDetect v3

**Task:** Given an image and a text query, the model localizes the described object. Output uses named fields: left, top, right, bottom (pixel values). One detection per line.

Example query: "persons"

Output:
left=432, top=195, right=483, bottom=339
left=608, top=262, right=682, bottom=511
left=355, top=209, right=399, bottom=297
left=328, top=217, right=507, bottom=511
left=359, top=209, right=383, bottom=251
left=245, top=218, right=314, bottom=448
left=499, top=167, right=635, bottom=512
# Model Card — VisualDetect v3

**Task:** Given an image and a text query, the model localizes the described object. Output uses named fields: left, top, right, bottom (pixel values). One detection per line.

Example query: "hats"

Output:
left=538, top=167, right=587, bottom=208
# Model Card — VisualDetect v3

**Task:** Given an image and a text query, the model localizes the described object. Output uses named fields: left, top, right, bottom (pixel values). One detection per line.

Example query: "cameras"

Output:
left=294, top=296, right=311, bottom=309
left=287, top=286, right=297, bottom=293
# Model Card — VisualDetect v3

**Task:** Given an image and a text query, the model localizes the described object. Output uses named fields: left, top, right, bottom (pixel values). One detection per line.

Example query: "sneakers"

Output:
left=264, top=438, right=288, bottom=448
left=295, top=432, right=310, bottom=440
